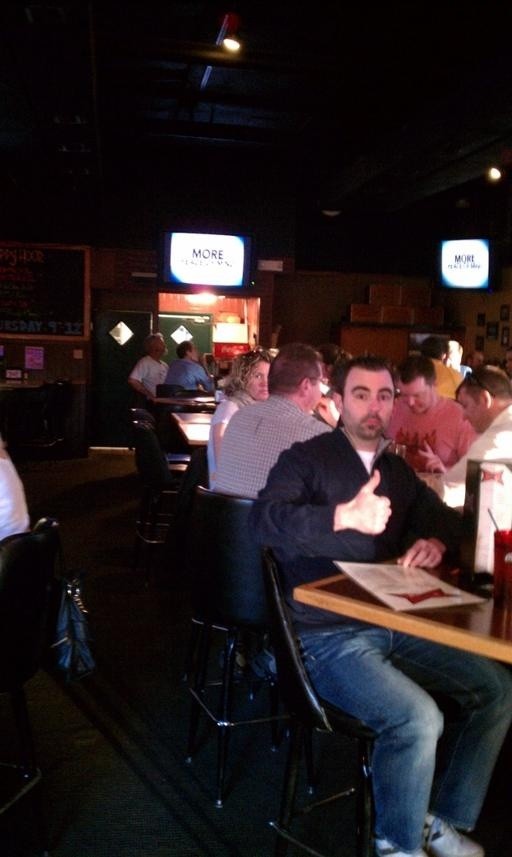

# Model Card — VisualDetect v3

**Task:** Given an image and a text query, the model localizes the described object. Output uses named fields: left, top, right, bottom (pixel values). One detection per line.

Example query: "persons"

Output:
left=391, top=354, right=479, bottom=472
left=1, top=429, right=33, bottom=605
left=217, top=342, right=333, bottom=496
left=419, top=336, right=511, bottom=400
left=313, top=343, right=353, bottom=428
left=126, top=330, right=169, bottom=400
left=209, top=347, right=277, bottom=492
left=445, top=366, right=512, bottom=575
left=162, top=340, right=213, bottom=394
left=243, top=352, right=511, bottom=857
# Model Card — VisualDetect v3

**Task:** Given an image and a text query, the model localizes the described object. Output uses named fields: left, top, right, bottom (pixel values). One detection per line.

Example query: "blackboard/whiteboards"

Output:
left=0, top=241, right=91, bottom=343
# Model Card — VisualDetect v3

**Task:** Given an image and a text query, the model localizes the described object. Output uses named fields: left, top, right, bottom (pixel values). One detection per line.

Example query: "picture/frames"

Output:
left=474, top=303, right=510, bottom=351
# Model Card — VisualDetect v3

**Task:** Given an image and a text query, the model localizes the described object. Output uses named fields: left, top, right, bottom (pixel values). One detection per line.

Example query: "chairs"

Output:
left=0, top=518, right=66, bottom=815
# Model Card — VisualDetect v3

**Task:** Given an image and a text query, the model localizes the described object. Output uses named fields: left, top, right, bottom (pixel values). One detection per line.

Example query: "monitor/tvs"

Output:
left=160, top=227, right=257, bottom=296
left=438, top=236, right=495, bottom=292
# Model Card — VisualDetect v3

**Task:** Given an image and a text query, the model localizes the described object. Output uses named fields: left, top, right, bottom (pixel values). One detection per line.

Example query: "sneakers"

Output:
left=420, top=809, right=489, bottom=857
left=371, top=833, right=432, bottom=857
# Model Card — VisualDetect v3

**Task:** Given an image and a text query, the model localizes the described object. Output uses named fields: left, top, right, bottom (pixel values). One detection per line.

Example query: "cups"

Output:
left=492, top=529, right=512, bottom=606
